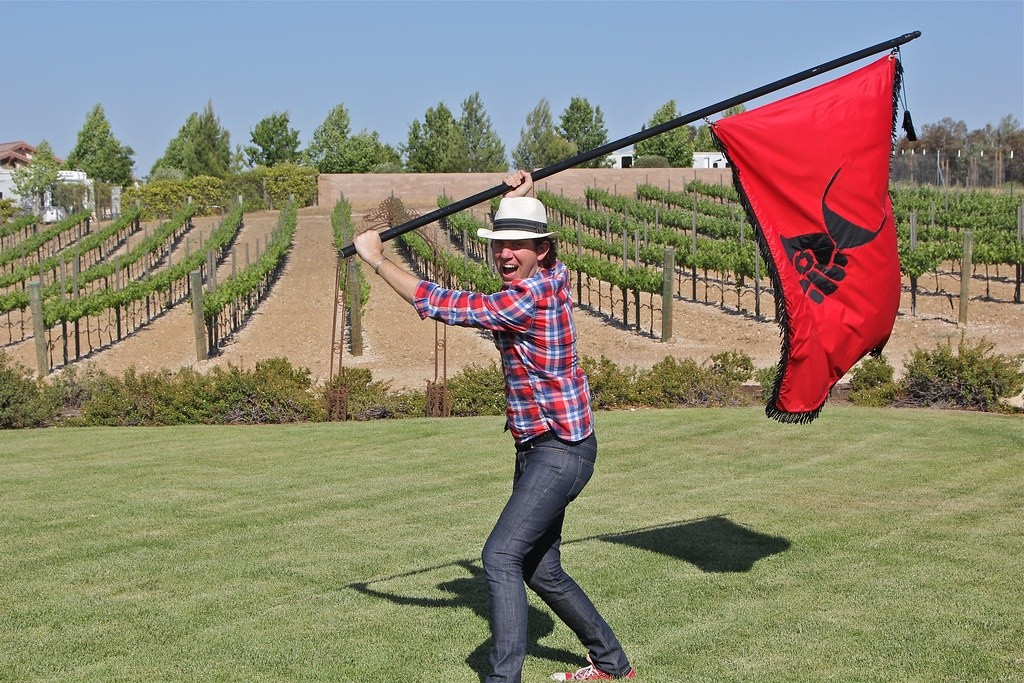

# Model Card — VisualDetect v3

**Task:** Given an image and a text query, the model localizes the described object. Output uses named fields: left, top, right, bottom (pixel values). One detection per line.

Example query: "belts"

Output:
left=514, top=430, right=557, bottom=452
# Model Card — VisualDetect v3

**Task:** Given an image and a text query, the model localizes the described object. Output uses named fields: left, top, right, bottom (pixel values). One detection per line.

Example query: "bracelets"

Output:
left=374, top=257, right=387, bottom=275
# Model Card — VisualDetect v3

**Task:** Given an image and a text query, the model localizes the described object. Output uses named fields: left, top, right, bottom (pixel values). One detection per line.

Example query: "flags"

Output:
left=712, top=55, right=903, bottom=426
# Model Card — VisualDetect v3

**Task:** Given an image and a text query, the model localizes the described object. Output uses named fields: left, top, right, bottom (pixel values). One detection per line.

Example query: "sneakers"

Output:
left=549, top=652, right=637, bottom=682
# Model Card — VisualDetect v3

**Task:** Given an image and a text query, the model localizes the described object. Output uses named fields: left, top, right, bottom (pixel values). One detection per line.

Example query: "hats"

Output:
left=477, top=197, right=558, bottom=240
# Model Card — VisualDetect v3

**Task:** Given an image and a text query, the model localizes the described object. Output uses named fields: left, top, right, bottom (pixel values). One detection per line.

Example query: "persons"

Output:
left=351, top=169, right=633, bottom=683
